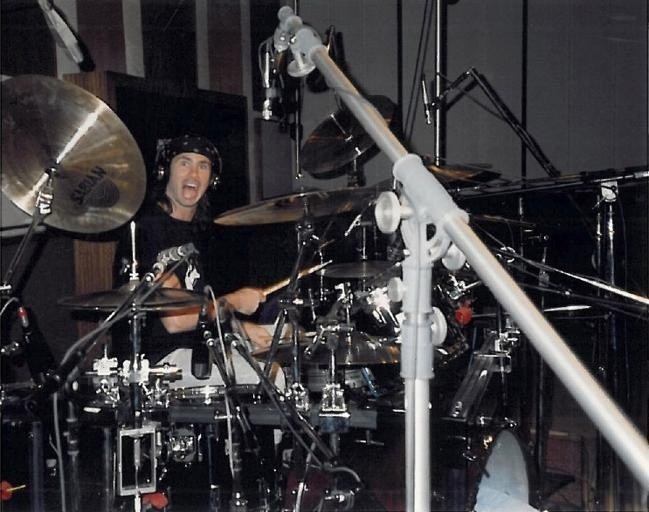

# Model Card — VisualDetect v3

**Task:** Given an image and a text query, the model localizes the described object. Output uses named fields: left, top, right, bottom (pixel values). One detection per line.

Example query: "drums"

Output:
left=1, top=362, right=177, bottom=511
left=176, top=382, right=292, bottom=512
left=430, top=416, right=543, bottom=511
left=327, top=260, right=470, bottom=403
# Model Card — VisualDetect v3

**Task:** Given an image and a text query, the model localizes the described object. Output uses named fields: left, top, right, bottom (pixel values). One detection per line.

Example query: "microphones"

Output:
left=191, top=292, right=214, bottom=381
left=19, top=307, right=44, bottom=384
left=261, top=42, right=275, bottom=121
left=215, top=299, right=232, bottom=369
left=152, top=243, right=193, bottom=263
left=38, top=0, right=96, bottom=72
left=420, top=80, right=433, bottom=125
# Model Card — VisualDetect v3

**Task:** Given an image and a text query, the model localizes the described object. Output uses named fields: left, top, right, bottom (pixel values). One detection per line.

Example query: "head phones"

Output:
left=151, top=135, right=224, bottom=192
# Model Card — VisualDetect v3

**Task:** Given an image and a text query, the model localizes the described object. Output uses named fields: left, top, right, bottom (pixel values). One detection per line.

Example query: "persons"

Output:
left=112, top=132, right=286, bottom=443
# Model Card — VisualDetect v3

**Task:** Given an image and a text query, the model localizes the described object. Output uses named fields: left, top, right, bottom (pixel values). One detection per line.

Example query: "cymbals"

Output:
left=369, top=162, right=491, bottom=192
left=59, top=284, right=202, bottom=312
left=1, top=74, right=147, bottom=235
left=215, top=187, right=385, bottom=226
left=316, top=257, right=391, bottom=279
left=253, top=335, right=404, bottom=367
left=301, top=93, right=396, bottom=176
